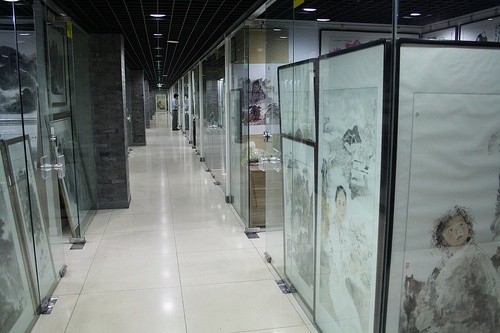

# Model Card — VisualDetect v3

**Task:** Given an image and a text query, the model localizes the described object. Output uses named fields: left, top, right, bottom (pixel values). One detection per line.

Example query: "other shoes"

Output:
left=172, top=129, right=180, bottom=131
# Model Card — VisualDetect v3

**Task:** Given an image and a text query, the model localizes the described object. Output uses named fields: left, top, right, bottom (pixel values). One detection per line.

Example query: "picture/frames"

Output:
left=275, top=58, right=317, bottom=148
left=379, top=37, right=500, bottom=333
left=0, top=22, right=72, bottom=124
left=0, top=138, right=40, bottom=333
left=311, top=39, right=389, bottom=332
left=4, top=134, right=58, bottom=312
left=44, top=111, right=94, bottom=239
left=279, top=132, right=318, bottom=315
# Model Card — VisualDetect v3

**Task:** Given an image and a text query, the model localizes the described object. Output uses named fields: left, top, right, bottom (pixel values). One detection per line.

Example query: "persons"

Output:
left=171, top=93, right=181, bottom=132
